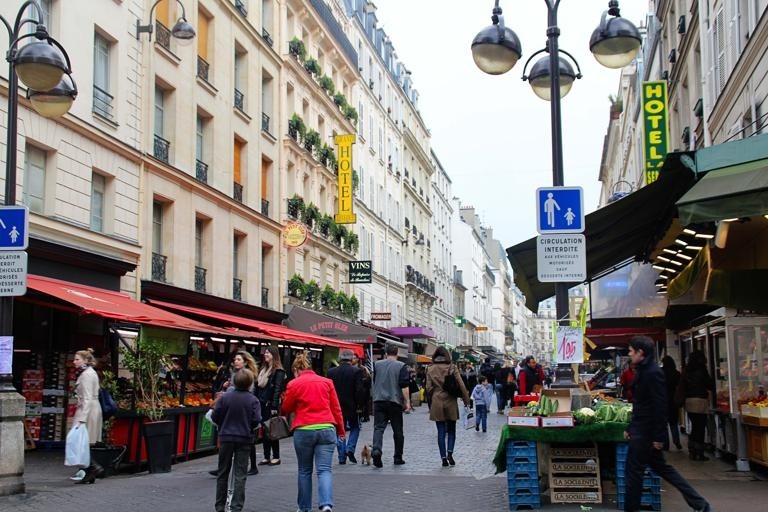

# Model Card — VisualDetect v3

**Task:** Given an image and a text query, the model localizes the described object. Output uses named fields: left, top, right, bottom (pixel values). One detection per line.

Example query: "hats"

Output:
left=340, top=350, right=353, bottom=360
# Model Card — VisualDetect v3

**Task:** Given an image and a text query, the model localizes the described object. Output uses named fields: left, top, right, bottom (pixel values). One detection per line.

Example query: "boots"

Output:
left=91, top=461, right=106, bottom=480
left=74, top=469, right=95, bottom=485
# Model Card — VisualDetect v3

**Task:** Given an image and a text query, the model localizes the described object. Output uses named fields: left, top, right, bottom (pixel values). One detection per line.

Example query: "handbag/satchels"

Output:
left=262, top=415, right=292, bottom=442
left=99, top=387, right=118, bottom=422
left=64, top=423, right=91, bottom=468
left=461, top=406, right=476, bottom=430
left=442, top=374, right=463, bottom=398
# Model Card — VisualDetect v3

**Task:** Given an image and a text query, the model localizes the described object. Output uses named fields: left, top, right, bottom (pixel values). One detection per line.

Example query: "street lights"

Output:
left=0, top=0, right=78, bottom=389
left=471, top=0, right=642, bottom=392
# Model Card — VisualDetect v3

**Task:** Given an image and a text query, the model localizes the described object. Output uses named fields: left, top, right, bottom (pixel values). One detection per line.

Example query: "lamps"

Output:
left=651, top=219, right=713, bottom=297
left=137, top=1, right=196, bottom=47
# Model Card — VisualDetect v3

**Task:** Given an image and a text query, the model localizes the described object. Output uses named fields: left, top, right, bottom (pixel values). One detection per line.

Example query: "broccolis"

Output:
left=573, top=403, right=633, bottom=423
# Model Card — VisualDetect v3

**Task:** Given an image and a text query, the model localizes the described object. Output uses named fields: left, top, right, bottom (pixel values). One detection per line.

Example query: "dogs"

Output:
left=361, top=445, right=373, bottom=465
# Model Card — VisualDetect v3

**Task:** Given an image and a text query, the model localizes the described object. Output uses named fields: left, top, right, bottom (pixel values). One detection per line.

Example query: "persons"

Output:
left=461, top=355, right=545, bottom=433
left=425, top=345, right=471, bottom=467
left=207, top=349, right=260, bottom=477
left=326, top=350, right=370, bottom=465
left=258, top=344, right=287, bottom=466
left=679, top=349, right=716, bottom=461
left=211, top=366, right=263, bottom=512
left=281, top=349, right=347, bottom=512
left=71, top=346, right=104, bottom=486
left=352, top=354, right=372, bottom=422
left=659, top=355, right=683, bottom=450
left=619, top=360, right=636, bottom=401
left=621, top=334, right=711, bottom=512
left=371, top=344, right=411, bottom=468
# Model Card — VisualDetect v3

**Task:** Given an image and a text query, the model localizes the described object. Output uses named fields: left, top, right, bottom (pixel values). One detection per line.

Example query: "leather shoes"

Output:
left=208, top=469, right=219, bottom=476
left=268, top=460, right=281, bottom=466
left=258, top=460, right=270, bottom=465
left=247, top=468, right=258, bottom=476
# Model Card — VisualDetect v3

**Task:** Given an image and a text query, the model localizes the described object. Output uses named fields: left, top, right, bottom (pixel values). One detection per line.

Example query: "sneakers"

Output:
left=688, top=454, right=696, bottom=460
left=321, top=506, right=332, bottom=512
left=394, top=460, right=405, bottom=465
left=441, top=457, right=455, bottom=466
left=694, top=501, right=710, bottom=512
left=695, top=454, right=710, bottom=462
left=339, top=451, right=357, bottom=465
left=371, top=449, right=383, bottom=468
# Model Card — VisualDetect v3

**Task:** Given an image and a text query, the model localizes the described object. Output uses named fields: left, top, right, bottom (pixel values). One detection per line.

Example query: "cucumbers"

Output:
left=523, top=394, right=559, bottom=418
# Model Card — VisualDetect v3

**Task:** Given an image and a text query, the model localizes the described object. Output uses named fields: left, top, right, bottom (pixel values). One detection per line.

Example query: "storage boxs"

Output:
left=22, top=345, right=86, bottom=450
left=504, top=385, right=662, bottom=512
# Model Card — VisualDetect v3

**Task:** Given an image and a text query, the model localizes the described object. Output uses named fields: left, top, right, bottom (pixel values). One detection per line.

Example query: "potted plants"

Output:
left=121, top=335, right=175, bottom=473
left=90, top=372, right=126, bottom=476
